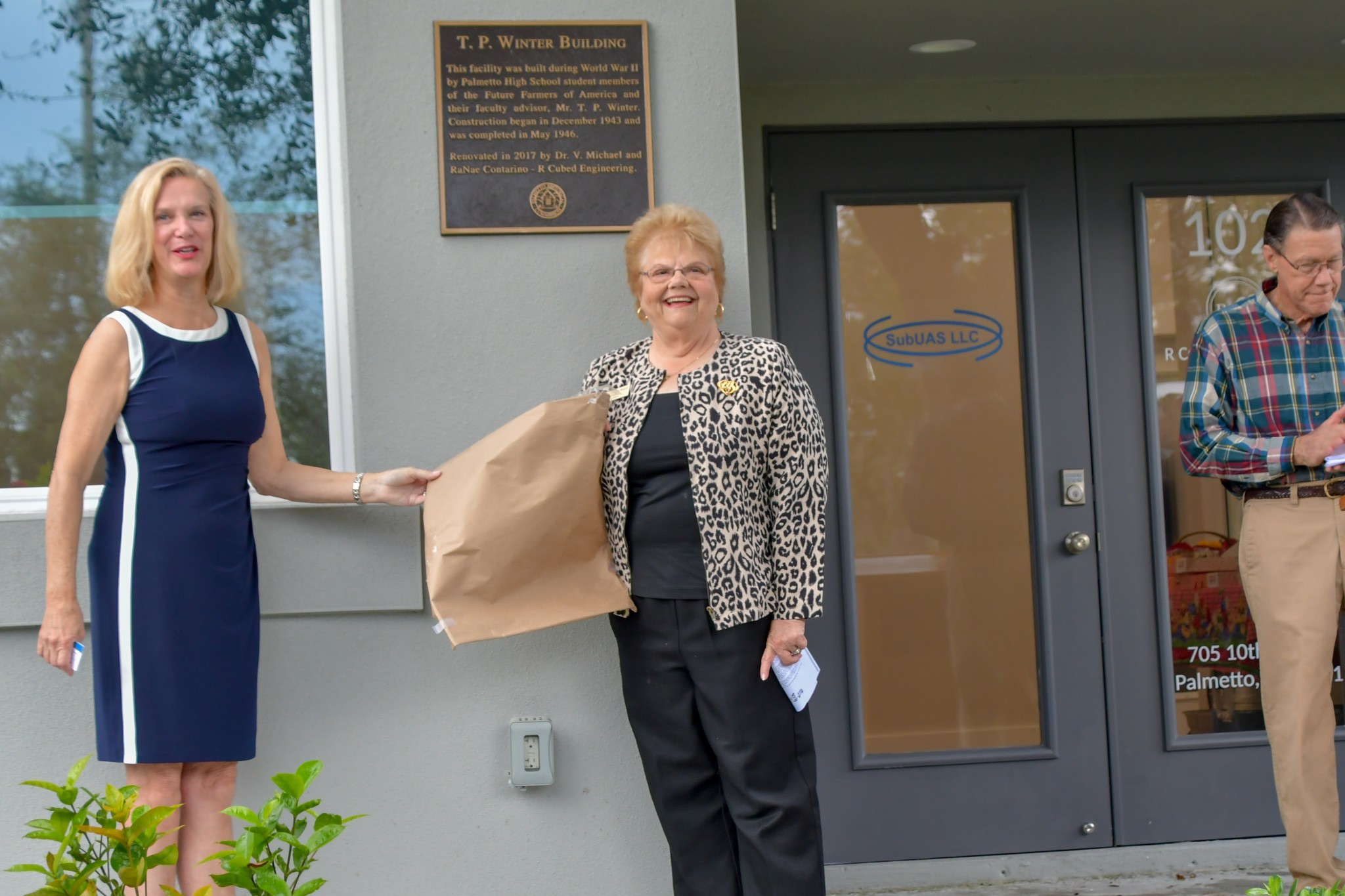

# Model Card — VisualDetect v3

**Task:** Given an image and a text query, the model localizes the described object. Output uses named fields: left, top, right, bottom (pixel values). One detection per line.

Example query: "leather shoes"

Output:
left=1237, top=480, right=1345, bottom=502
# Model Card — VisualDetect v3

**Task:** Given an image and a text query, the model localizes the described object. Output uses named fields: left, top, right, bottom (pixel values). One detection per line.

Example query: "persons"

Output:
left=569, top=202, right=835, bottom=896
left=1177, top=190, right=1345, bottom=894
left=35, top=151, right=446, bottom=895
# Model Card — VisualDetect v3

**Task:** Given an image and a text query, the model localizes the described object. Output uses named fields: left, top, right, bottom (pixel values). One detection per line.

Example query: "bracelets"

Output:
left=352, top=472, right=367, bottom=505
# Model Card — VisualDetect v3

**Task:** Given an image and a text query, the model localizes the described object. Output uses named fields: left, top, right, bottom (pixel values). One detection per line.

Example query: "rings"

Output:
left=790, top=647, right=801, bottom=657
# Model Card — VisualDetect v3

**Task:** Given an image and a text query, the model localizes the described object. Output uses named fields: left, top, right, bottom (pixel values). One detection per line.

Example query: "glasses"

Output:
left=1277, top=250, right=1344, bottom=275
left=637, top=260, right=714, bottom=283
